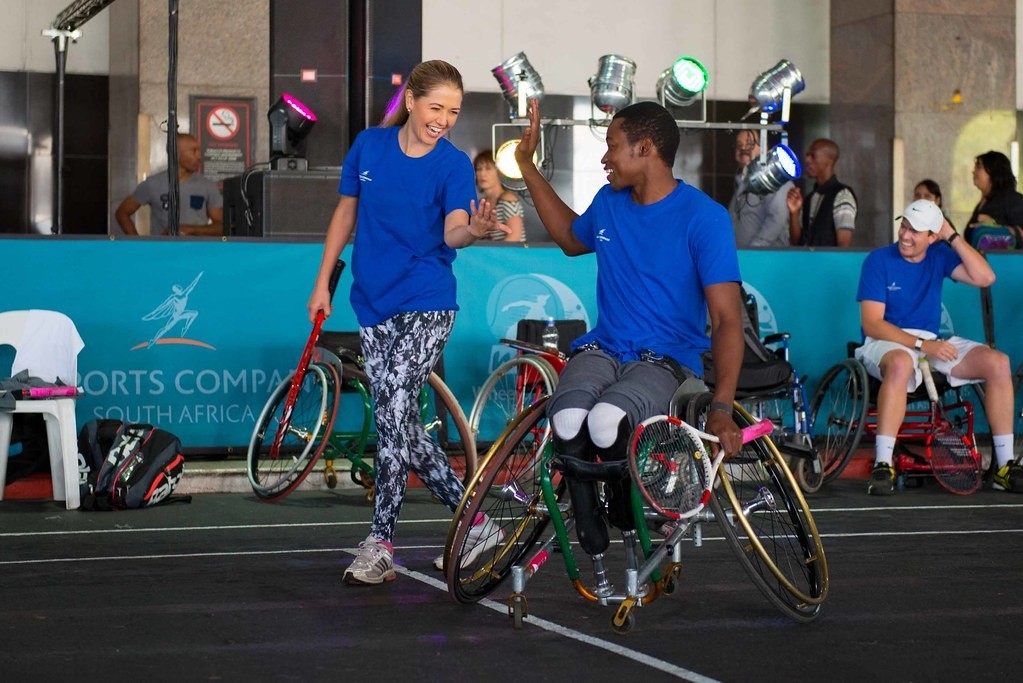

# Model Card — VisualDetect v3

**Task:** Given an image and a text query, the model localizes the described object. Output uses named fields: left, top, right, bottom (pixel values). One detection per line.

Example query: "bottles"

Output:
left=542, top=321, right=559, bottom=350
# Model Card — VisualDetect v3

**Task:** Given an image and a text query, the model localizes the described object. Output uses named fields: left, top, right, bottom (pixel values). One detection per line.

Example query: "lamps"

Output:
left=749, top=59, right=805, bottom=115
left=656, top=56, right=709, bottom=108
left=587, top=54, right=638, bottom=112
left=493, top=140, right=539, bottom=192
left=733, top=144, right=802, bottom=194
left=491, top=51, right=544, bottom=110
left=267, top=92, right=316, bottom=144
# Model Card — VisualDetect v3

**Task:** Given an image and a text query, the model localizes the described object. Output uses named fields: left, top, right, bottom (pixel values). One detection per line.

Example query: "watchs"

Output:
left=915, top=338, right=924, bottom=351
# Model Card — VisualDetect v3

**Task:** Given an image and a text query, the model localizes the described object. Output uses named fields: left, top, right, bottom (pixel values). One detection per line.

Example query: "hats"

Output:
left=896, top=198, right=943, bottom=233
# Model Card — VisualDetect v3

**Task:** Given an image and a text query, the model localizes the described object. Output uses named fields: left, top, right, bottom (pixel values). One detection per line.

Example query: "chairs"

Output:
left=0, top=309, right=80, bottom=509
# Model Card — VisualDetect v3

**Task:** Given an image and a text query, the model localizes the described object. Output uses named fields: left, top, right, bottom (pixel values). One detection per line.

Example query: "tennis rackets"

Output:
left=625, top=415, right=774, bottom=522
left=919, top=357, right=984, bottom=496
left=268, top=258, right=346, bottom=461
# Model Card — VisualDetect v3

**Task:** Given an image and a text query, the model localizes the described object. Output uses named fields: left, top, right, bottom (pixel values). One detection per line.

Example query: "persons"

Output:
left=855, top=199, right=1023, bottom=494
left=473, top=150, right=527, bottom=242
left=114, top=134, right=224, bottom=237
left=307, top=59, right=513, bottom=584
left=514, top=98, right=744, bottom=597
left=913, top=180, right=942, bottom=208
left=728, top=129, right=802, bottom=247
left=786, top=138, right=857, bottom=247
left=964, top=151, right=1023, bottom=248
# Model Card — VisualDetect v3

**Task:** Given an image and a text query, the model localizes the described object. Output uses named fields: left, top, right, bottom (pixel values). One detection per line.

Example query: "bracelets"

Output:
left=947, top=233, right=959, bottom=244
left=711, top=402, right=733, bottom=415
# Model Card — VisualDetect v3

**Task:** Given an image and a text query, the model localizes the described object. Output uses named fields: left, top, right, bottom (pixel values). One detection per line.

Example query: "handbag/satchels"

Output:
left=78, top=419, right=192, bottom=511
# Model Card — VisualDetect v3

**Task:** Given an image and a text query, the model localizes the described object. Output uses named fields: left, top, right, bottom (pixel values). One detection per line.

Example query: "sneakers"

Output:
left=344, top=536, right=398, bottom=586
left=992, top=460, right=1023, bottom=496
left=433, top=512, right=507, bottom=572
left=866, top=461, right=898, bottom=494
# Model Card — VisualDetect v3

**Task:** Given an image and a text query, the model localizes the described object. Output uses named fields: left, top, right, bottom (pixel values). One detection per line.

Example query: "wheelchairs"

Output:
left=245, top=322, right=480, bottom=504
left=795, top=322, right=1001, bottom=495
left=441, top=351, right=831, bottom=634
left=470, top=319, right=680, bottom=516
left=674, top=280, right=827, bottom=497
